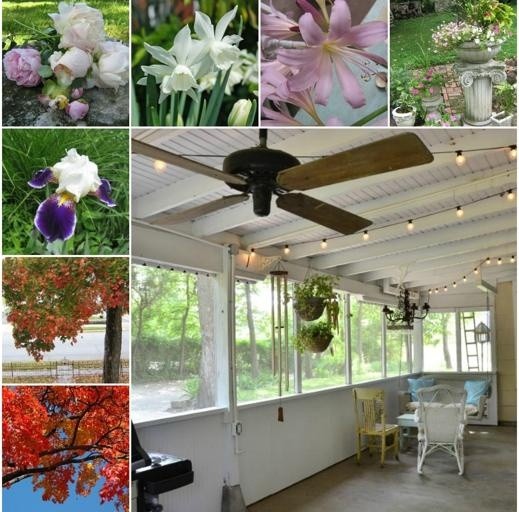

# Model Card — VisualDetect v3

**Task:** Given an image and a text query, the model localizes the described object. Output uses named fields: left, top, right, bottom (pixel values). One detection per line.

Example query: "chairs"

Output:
left=412, top=383, right=469, bottom=477
left=349, top=385, right=400, bottom=468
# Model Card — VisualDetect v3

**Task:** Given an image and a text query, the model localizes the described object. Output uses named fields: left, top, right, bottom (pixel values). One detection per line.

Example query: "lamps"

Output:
left=380, top=284, right=431, bottom=328
left=472, top=322, right=491, bottom=371
left=447, top=143, right=517, bottom=167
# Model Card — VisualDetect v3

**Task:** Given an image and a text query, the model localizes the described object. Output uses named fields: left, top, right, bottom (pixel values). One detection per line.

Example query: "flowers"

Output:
left=393, top=1, right=517, bottom=129
left=257, top=0, right=387, bottom=127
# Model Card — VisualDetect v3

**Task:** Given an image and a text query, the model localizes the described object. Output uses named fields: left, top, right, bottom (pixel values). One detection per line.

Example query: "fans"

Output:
left=129, top=129, right=437, bottom=241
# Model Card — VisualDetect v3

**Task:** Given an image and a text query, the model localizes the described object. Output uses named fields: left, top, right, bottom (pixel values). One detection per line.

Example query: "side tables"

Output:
left=396, top=411, right=419, bottom=450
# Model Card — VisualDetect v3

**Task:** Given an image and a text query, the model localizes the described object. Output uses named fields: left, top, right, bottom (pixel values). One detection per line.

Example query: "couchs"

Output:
left=397, top=372, right=497, bottom=426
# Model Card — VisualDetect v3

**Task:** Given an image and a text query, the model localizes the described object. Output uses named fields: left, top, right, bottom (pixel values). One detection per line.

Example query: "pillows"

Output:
left=461, top=379, right=490, bottom=406
left=407, top=377, right=439, bottom=403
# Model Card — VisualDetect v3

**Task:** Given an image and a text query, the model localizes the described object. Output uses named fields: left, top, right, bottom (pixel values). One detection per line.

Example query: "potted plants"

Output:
left=283, top=273, right=343, bottom=356
